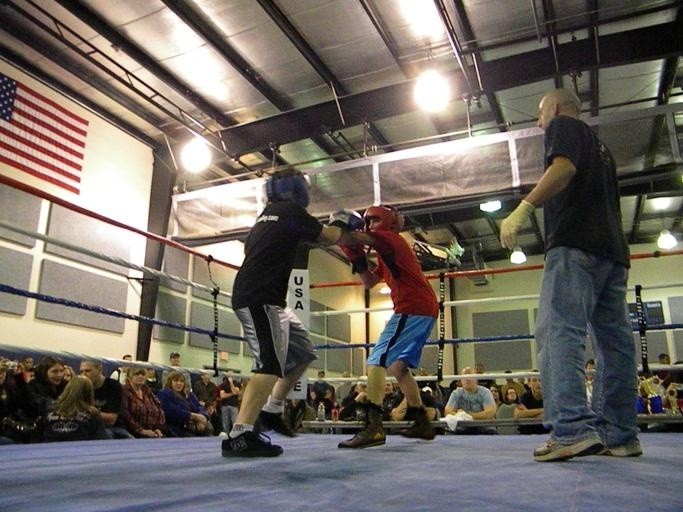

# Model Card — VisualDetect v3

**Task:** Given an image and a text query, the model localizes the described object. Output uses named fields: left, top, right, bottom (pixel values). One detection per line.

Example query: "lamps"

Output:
left=413, top=42, right=450, bottom=112
left=507, top=235, right=527, bottom=266
left=656, top=215, right=678, bottom=250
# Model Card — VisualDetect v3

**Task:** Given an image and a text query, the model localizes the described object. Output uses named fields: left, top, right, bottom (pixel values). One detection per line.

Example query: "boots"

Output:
left=338, top=402, right=385, bottom=448
left=399, top=402, right=435, bottom=440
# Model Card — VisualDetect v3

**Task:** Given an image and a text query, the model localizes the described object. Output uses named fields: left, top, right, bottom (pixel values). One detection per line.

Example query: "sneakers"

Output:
left=534, top=438, right=642, bottom=461
left=222, top=424, right=283, bottom=457
left=259, top=409, right=296, bottom=437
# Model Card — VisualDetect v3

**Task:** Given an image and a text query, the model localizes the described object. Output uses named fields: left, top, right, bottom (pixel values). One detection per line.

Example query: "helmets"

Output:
left=265, top=172, right=309, bottom=207
left=364, top=205, right=404, bottom=234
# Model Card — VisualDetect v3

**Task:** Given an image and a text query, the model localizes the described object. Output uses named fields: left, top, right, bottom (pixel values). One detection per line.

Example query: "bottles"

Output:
left=331, top=401, right=338, bottom=421
left=317, top=402, right=325, bottom=422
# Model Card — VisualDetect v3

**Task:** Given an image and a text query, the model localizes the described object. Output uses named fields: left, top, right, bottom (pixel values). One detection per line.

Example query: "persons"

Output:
left=0, top=351, right=332, bottom=444
left=498, top=86, right=646, bottom=462
left=219, top=164, right=377, bottom=460
left=337, top=363, right=502, bottom=434
left=653, top=354, right=683, bottom=399
left=336, top=203, right=441, bottom=454
left=499, top=370, right=544, bottom=416
left=585, top=359, right=595, bottom=404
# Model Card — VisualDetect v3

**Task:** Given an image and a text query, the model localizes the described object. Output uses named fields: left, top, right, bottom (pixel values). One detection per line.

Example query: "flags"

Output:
left=0, top=71, right=90, bottom=196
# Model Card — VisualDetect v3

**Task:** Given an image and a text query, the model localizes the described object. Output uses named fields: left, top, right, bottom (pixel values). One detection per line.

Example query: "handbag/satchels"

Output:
left=169, top=415, right=214, bottom=436
left=2, top=416, right=43, bottom=443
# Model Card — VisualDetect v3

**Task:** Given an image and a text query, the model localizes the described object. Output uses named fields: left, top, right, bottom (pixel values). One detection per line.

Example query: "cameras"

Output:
left=6, top=360, right=18, bottom=370
left=667, top=388, right=677, bottom=397
left=652, top=375, right=664, bottom=388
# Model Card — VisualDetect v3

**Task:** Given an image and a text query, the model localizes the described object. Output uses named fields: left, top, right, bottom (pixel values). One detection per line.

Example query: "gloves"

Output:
left=329, top=209, right=365, bottom=231
left=339, top=242, right=365, bottom=261
left=500, top=198, right=535, bottom=251
